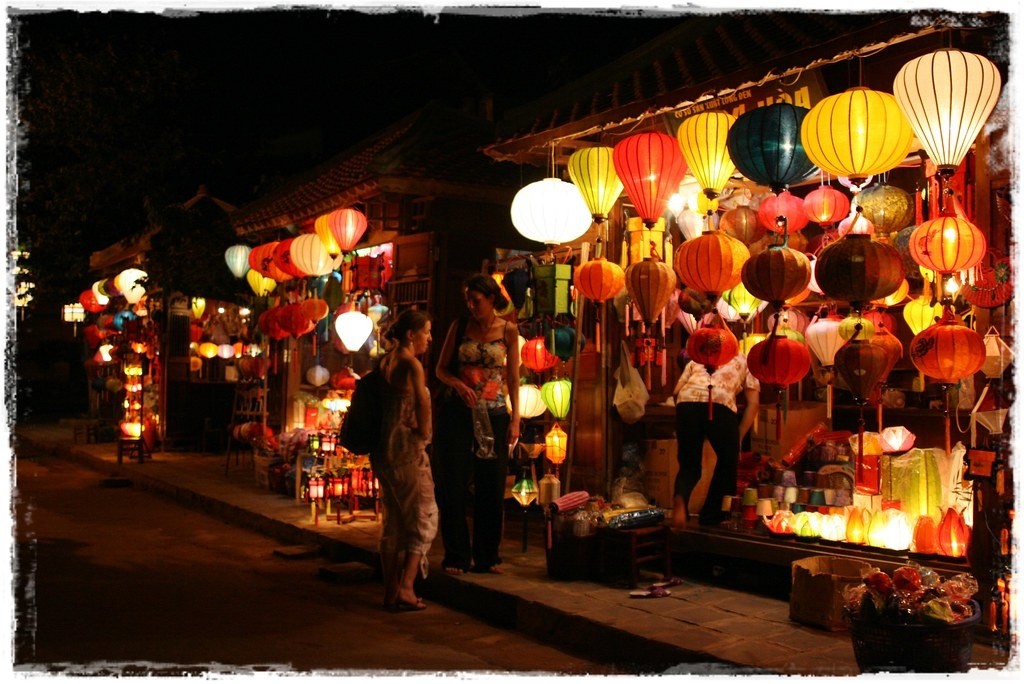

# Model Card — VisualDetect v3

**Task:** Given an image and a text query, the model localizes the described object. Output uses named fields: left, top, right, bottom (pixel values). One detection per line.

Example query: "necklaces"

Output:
left=482, top=316, right=496, bottom=337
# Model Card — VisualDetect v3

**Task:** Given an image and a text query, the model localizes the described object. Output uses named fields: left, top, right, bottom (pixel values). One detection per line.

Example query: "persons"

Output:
left=378, top=310, right=438, bottom=608
left=673, top=350, right=760, bottom=524
left=436, top=275, right=520, bottom=576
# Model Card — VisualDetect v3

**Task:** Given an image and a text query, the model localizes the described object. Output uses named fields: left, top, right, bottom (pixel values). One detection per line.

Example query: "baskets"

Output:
left=845, top=597, right=981, bottom=673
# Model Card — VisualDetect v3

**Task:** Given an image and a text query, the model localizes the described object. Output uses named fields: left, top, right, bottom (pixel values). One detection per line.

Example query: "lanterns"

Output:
left=573, top=173, right=989, bottom=566
left=510, top=178, right=593, bottom=246
left=257, top=254, right=390, bottom=523
left=63, top=268, right=156, bottom=452
left=800, top=87, right=913, bottom=186
left=503, top=261, right=586, bottom=553
left=225, top=207, right=367, bottom=296
left=190, top=297, right=273, bottom=449
left=567, top=146, right=624, bottom=223
left=893, top=48, right=1002, bottom=182
left=613, top=131, right=686, bottom=230
left=727, top=103, right=819, bottom=195
left=677, top=109, right=738, bottom=200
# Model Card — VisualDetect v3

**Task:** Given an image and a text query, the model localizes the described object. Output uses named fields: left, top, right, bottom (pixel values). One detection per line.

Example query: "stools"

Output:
left=70, top=419, right=101, bottom=445
left=118, top=436, right=147, bottom=464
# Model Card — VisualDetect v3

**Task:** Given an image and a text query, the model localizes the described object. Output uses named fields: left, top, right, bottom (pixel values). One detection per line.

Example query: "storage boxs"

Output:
left=789, top=556, right=870, bottom=632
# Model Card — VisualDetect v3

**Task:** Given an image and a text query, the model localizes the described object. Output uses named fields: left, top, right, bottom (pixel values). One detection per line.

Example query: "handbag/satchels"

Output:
left=339, top=353, right=394, bottom=456
left=609, top=339, right=651, bottom=424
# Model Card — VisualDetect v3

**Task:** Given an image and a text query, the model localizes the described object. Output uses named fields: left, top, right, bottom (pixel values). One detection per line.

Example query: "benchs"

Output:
left=563, top=518, right=671, bottom=590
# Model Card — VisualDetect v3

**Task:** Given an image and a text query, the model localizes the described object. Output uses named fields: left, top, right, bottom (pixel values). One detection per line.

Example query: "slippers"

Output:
left=628, top=586, right=671, bottom=597
left=398, top=596, right=426, bottom=610
left=648, top=575, right=684, bottom=589
left=472, top=560, right=504, bottom=574
left=382, top=602, right=400, bottom=610
left=444, top=559, right=471, bottom=574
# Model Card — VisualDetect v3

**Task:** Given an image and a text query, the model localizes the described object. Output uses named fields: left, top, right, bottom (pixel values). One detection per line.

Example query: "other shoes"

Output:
left=699, top=511, right=731, bottom=525
